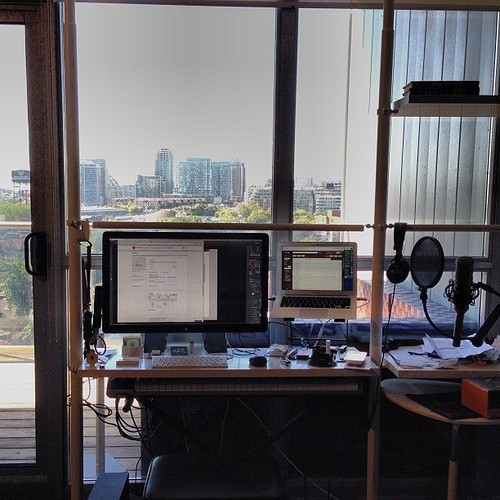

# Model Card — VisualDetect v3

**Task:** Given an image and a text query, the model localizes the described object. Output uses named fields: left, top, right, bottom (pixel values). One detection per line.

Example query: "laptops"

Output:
left=269, top=241, right=358, bottom=320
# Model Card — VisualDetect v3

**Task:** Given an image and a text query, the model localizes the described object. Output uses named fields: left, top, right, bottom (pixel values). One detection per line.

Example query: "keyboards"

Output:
left=152, top=356, right=228, bottom=368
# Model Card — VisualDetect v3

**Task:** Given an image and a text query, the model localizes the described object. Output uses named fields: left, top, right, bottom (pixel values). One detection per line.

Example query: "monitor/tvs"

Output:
left=102, top=230, right=269, bottom=333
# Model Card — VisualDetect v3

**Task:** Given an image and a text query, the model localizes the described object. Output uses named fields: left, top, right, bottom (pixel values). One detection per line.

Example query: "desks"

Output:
left=379, top=378, right=500, bottom=500
left=75, top=348, right=381, bottom=500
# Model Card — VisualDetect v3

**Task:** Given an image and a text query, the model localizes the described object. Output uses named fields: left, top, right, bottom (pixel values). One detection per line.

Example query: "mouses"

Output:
left=249, top=356, right=267, bottom=367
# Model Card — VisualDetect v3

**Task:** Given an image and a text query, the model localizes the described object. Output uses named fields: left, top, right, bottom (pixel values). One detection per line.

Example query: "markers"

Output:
left=287, top=348, right=297, bottom=357
left=326, top=340, right=330, bottom=354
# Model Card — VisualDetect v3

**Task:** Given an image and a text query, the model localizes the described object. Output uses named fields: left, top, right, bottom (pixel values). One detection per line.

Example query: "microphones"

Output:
left=92, top=286, right=102, bottom=345
left=451, top=256, right=475, bottom=347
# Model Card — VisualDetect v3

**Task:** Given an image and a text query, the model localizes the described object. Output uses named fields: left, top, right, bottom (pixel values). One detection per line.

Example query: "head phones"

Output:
left=386, top=222, right=410, bottom=284
